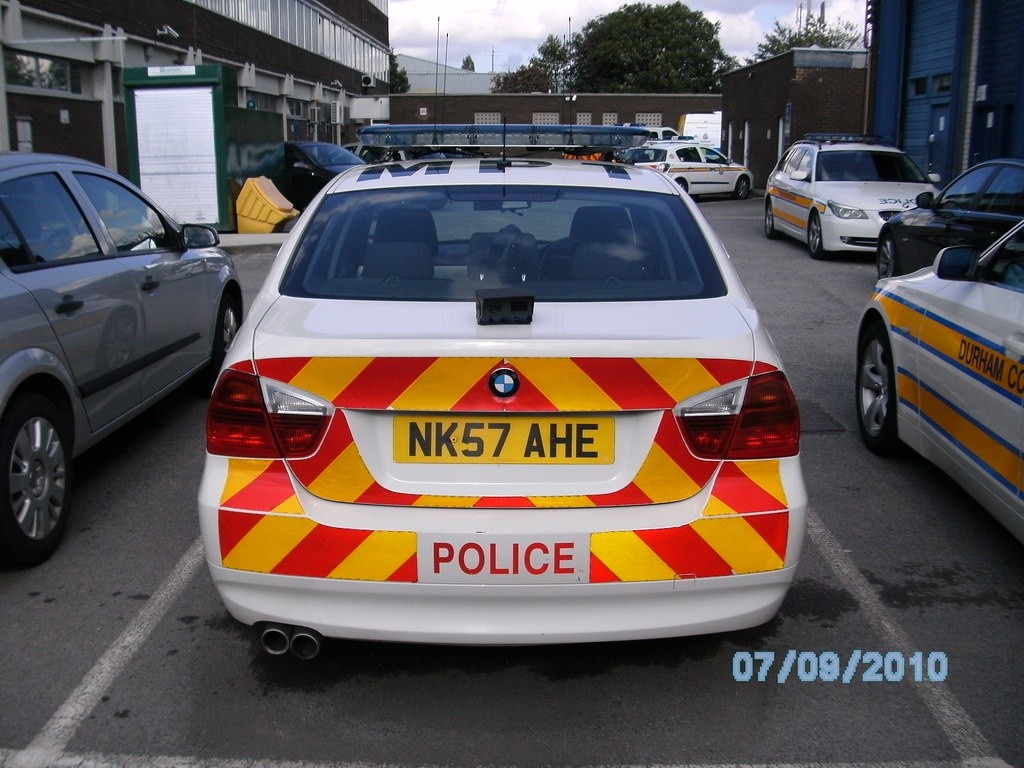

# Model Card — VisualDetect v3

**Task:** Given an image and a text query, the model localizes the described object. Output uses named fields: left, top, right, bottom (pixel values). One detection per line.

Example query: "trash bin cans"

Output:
left=236, top=175, right=301, bottom=234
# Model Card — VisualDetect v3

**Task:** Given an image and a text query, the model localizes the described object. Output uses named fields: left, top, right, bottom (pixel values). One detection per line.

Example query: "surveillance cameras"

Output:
left=164, top=24, right=179, bottom=38
left=334, top=80, right=342, bottom=87
left=572, top=94, right=577, bottom=101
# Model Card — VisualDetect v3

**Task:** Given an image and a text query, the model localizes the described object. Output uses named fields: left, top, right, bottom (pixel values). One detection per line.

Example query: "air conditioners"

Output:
left=330, top=100, right=344, bottom=125
left=361, top=74, right=376, bottom=88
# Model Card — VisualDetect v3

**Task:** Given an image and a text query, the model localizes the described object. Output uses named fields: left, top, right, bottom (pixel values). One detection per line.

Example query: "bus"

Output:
left=354, top=124, right=650, bottom=163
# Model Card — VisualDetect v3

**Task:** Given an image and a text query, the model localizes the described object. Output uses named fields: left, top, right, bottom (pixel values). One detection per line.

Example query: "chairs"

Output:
left=538, top=241, right=669, bottom=296
left=341, top=240, right=455, bottom=298
left=535, top=204, right=670, bottom=280
left=0, top=196, right=57, bottom=267
left=372, top=206, right=440, bottom=256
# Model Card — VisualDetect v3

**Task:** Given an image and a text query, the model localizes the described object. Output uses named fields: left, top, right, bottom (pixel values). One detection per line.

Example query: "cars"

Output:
left=0, top=148, right=246, bottom=572
left=762, top=132, right=948, bottom=261
left=251, top=141, right=368, bottom=213
left=623, top=135, right=756, bottom=201
left=639, top=127, right=681, bottom=141
left=875, top=158, right=1024, bottom=281
left=854, top=215, right=1023, bottom=546
left=195, top=159, right=809, bottom=667
